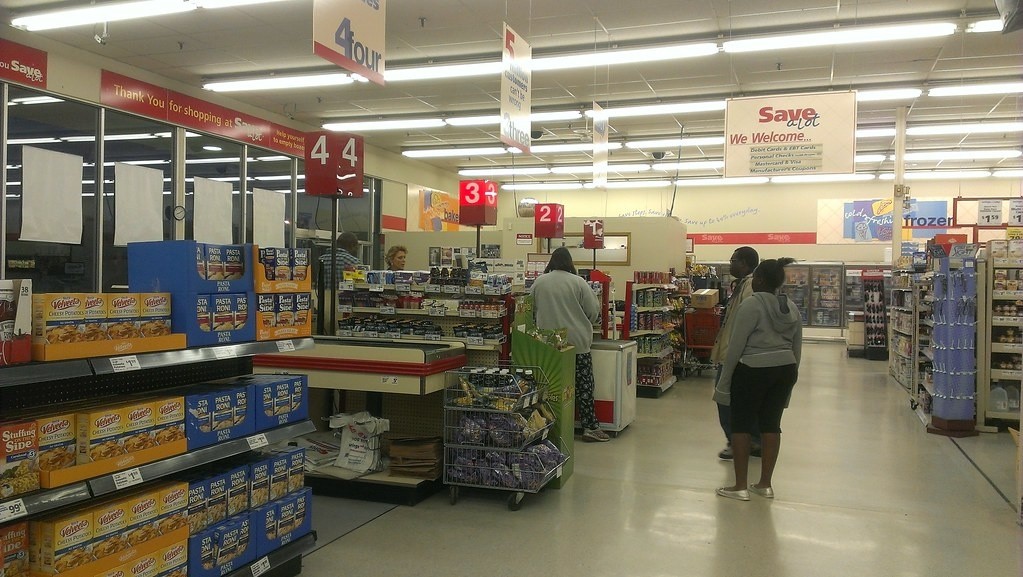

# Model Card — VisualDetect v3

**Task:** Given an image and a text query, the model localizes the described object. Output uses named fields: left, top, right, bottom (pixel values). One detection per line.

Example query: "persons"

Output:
left=384, top=246, right=407, bottom=271
left=529, top=247, right=610, bottom=442
left=319, top=232, right=363, bottom=290
left=711, top=258, right=802, bottom=501
left=710, top=246, right=762, bottom=460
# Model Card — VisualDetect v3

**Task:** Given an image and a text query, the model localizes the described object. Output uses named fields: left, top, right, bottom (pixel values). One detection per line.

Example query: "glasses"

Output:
left=729, top=257, right=746, bottom=264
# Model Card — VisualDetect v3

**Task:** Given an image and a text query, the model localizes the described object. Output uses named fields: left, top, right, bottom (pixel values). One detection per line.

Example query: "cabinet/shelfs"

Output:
left=862, top=258, right=1023, bottom=438
left=253, top=267, right=679, bottom=505
left=0, top=337, right=317, bottom=577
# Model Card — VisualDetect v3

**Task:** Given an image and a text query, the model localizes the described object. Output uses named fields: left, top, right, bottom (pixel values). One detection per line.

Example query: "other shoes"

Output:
left=716, top=487, right=750, bottom=501
left=719, top=447, right=735, bottom=461
left=750, top=448, right=763, bottom=458
left=749, top=483, right=775, bottom=498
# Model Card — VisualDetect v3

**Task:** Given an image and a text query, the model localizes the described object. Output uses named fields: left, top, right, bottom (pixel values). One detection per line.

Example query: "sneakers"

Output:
left=583, top=428, right=611, bottom=442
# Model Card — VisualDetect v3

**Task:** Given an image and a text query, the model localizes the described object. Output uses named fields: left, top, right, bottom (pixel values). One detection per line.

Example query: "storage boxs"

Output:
left=0, top=241, right=311, bottom=577
left=691, top=288, right=720, bottom=309
left=987, top=239, right=1023, bottom=258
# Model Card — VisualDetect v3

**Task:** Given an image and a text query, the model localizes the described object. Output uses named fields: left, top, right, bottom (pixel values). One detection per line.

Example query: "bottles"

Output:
left=457, top=301, right=505, bottom=312
left=468, top=367, right=537, bottom=395
left=430, top=267, right=469, bottom=286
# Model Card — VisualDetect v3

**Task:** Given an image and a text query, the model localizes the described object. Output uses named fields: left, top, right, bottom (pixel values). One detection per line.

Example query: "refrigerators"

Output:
left=575, top=339, right=636, bottom=438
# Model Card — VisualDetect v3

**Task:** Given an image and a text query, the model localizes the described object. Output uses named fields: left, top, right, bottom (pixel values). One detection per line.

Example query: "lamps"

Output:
left=8, top=0, right=1023, bottom=191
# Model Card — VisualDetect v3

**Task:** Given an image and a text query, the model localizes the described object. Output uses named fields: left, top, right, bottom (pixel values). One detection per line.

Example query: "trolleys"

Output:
left=682, top=307, right=723, bottom=378
